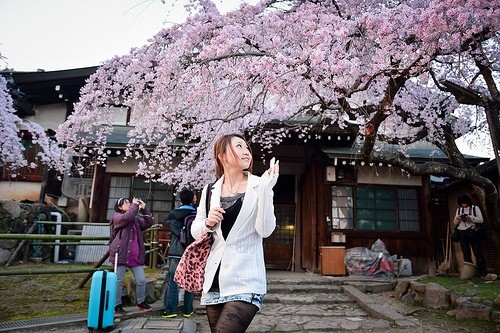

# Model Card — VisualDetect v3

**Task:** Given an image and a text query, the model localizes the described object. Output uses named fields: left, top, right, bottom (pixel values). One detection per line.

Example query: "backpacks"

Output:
left=180, top=208, right=202, bottom=247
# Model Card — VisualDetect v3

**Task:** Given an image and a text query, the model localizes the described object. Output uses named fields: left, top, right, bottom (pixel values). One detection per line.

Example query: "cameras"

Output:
left=459, top=214, right=468, bottom=222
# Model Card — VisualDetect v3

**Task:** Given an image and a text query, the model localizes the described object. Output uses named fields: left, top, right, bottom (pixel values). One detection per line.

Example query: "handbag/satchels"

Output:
left=173, top=181, right=214, bottom=295
left=450, top=229, right=460, bottom=242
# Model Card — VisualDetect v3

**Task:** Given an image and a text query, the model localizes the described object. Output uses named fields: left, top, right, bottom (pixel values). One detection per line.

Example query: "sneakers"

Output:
left=137, top=302, right=152, bottom=311
left=114, top=305, right=128, bottom=314
left=162, top=310, right=177, bottom=317
left=184, top=310, right=194, bottom=318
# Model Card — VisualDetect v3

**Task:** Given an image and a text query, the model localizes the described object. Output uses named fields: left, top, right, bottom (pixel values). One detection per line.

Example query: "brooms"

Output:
left=436, top=221, right=449, bottom=273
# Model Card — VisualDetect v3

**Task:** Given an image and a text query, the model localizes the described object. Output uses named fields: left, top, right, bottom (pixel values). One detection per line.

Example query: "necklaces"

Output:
left=224, top=175, right=244, bottom=195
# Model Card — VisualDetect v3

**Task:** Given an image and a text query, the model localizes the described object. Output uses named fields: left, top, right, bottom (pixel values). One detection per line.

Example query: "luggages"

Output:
left=87, top=251, right=119, bottom=332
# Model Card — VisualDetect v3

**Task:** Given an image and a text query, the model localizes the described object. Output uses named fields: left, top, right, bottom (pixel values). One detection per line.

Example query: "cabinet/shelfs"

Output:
left=319, top=246, right=346, bottom=277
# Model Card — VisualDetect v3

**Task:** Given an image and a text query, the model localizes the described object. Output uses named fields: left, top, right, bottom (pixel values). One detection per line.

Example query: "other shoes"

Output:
left=480, top=275, right=485, bottom=279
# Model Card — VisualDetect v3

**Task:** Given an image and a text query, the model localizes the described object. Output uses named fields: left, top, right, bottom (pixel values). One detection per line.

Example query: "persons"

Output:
left=454, top=194, right=487, bottom=277
left=162, top=189, right=197, bottom=318
left=109, top=197, right=155, bottom=314
left=190, top=134, right=279, bottom=333
